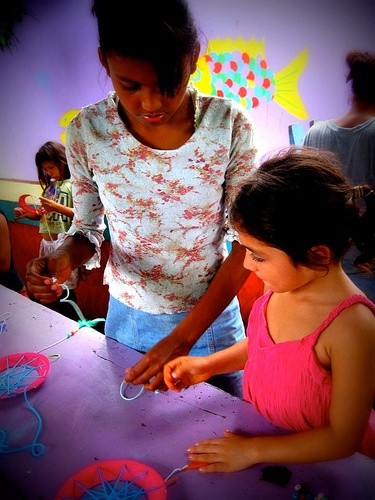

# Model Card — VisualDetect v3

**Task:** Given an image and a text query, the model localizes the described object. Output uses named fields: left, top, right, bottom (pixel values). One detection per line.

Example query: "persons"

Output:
left=301, top=50, right=375, bottom=249
left=25, top=1, right=247, bottom=402
left=13, top=142, right=79, bottom=290
left=163, top=146, right=375, bottom=473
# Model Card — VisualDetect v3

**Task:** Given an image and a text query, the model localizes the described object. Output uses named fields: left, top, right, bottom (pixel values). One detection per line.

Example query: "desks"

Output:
left=0, top=285, right=375, bottom=500
